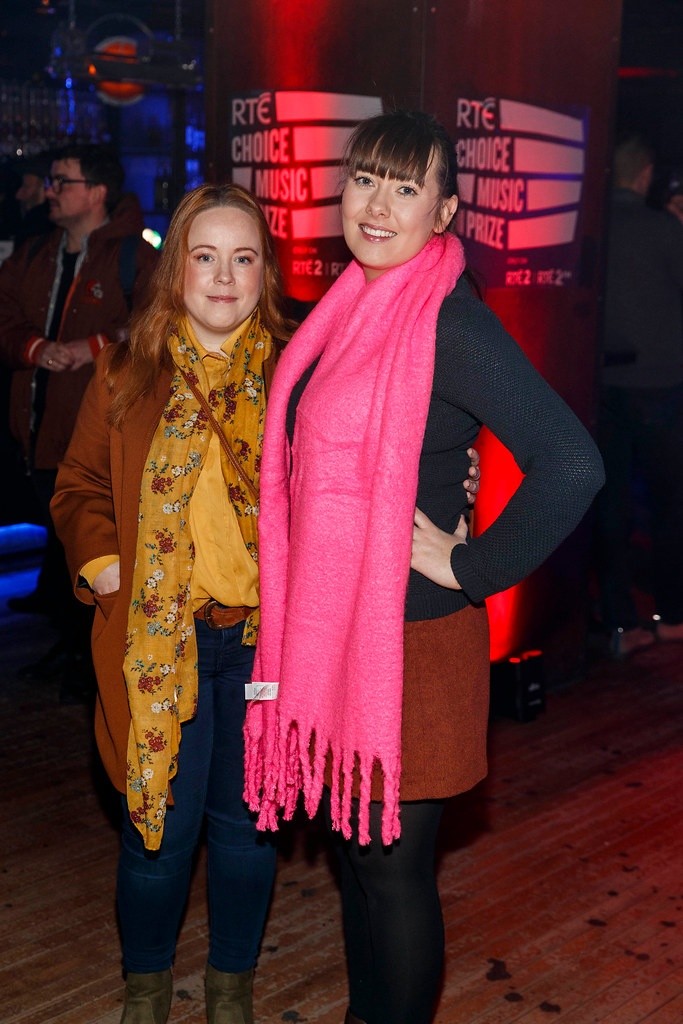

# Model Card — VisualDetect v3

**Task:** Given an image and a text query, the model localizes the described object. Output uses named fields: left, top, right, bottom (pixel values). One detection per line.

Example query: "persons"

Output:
left=241, top=110, right=607, bottom=1023
left=0, top=143, right=161, bottom=706
left=596, top=133, right=683, bottom=664
left=49, top=182, right=289, bottom=1024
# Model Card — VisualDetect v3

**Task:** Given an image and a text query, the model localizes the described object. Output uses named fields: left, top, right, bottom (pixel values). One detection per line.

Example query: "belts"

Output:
left=194, top=601, right=259, bottom=630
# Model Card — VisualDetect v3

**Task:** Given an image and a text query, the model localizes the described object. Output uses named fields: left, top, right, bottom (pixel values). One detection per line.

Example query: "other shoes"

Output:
left=16, top=651, right=62, bottom=682
left=656, top=623, right=683, bottom=641
left=59, top=679, right=91, bottom=705
left=7, top=590, right=53, bottom=614
left=608, top=625, right=656, bottom=660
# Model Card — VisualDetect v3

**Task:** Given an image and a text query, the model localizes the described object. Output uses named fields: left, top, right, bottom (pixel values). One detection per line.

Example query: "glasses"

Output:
left=45, top=175, right=98, bottom=194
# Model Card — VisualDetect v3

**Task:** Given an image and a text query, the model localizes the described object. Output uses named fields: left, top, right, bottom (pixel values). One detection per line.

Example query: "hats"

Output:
left=24, top=150, right=67, bottom=178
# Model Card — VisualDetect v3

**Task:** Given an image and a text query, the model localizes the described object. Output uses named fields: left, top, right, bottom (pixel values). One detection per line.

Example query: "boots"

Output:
left=120, top=969, right=173, bottom=1024
left=205, top=962, right=254, bottom=1024
